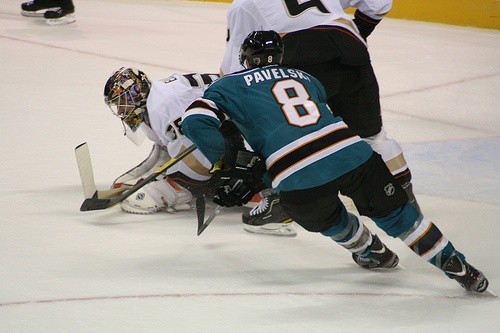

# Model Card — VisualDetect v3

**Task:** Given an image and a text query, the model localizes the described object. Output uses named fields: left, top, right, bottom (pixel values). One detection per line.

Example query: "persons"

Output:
left=19, top=0, right=77, bottom=26
left=74, top=67, right=265, bottom=215
left=181, top=30, right=497, bottom=297
left=219, top=0, right=425, bottom=236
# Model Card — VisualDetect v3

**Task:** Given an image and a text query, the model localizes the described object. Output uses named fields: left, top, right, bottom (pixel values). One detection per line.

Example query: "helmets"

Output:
left=104, top=67, right=151, bottom=133
left=240, top=30, right=284, bottom=64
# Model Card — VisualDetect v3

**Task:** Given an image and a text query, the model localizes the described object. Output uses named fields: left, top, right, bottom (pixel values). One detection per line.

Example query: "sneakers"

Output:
left=44, top=8, right=76, bottom=26
left=21, top=0, right=61, bottom=17
left=442, top=250, right=497, bottom=297
left=352, top=234, right=404, bottom=270
left=243, top=193, right=296, bottom=234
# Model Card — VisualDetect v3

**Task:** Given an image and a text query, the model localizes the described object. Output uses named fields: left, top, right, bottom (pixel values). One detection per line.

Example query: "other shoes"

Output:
left=402, top=183, right=422, bottom=216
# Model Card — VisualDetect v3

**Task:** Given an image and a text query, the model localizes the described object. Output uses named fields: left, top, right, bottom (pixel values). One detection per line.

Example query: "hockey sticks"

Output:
left=80, top=144, right=197, bottom=211
left=75, top=141, right=129, bottom=200
left=195, top=158, right=260, bottom=237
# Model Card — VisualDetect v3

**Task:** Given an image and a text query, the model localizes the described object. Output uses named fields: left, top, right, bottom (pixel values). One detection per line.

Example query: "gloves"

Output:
left=208, top=160, right=254, bottom=207
